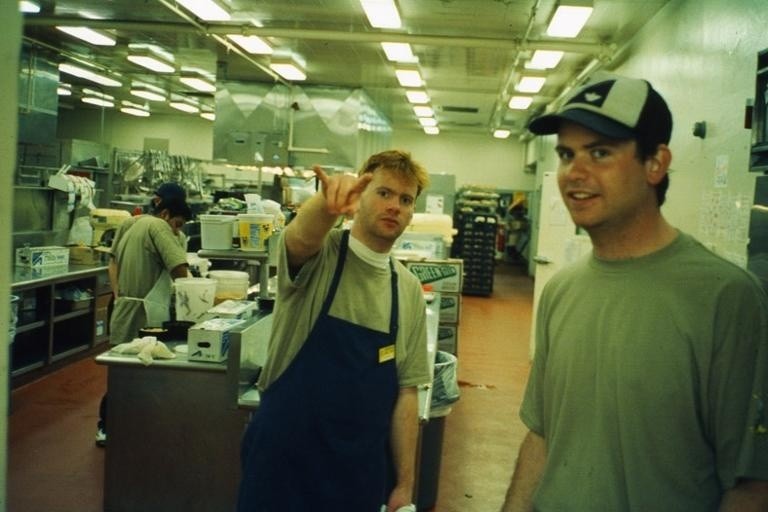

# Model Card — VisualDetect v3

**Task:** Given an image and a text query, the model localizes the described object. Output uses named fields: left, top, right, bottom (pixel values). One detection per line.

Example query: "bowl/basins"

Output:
left=258, top=298, right=274, bottom=311
left=138, top=326, right=169, bottom=342
left=163, top=320, right=195, bottom=341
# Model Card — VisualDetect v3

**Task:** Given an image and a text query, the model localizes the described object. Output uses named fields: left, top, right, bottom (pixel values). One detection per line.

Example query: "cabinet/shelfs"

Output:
left=94, top=338, right=250, bottom=512
left=11, top=270, right=112, bottom=392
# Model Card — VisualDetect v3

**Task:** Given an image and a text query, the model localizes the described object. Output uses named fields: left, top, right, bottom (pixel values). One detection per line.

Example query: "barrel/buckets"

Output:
left=209, top=270, right=249, bottom=306
left=201, top=215, right=234, bottom=250
left=238, top=215, right=272, bottom=252
left=176, top=279, right=217, bottom=327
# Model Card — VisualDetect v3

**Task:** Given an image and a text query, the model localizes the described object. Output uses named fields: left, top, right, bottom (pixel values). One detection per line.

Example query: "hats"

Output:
left=153, top=183, right=186, bottom=204
left=524, top=79, right=671, bottom=149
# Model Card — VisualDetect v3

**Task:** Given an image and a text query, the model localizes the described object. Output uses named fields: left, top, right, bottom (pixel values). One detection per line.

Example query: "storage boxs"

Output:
left=188, top=319, right=247, bottom=363
left=441, top=294, right=460, bottom=324
left=408, top=261, right=464, bottom=295
left=16, top=246, right=70, bottom=267
left=397, top=232, right=453, bottom=261
left=96, top=294, right=112, bottom=339
left=438, top=324, right=459, bottom=355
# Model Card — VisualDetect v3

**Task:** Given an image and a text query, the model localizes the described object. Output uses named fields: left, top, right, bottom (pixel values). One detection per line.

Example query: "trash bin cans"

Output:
left=418, top=349, right=459, bottom=512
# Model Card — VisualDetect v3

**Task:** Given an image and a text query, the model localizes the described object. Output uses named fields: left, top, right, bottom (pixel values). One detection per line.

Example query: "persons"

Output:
left=500, top=68, right=767, bottom=511
left=234, top=148, right=431, bottom=512
left=107, top=183, right=187, bottom=336
left=96, top=197, right=190, bottom=448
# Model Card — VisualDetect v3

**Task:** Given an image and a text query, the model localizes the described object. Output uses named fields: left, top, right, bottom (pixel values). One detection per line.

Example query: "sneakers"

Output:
left=95, top=424, right=108, bottom=444
